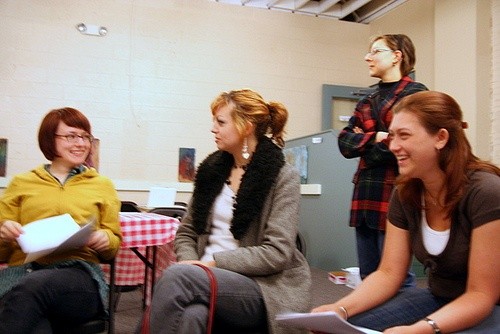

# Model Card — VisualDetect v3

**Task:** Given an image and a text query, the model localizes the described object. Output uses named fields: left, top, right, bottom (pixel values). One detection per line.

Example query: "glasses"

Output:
left=56, top=133, right=92, bottom=142
left=369, top=47, right=392, bottom=54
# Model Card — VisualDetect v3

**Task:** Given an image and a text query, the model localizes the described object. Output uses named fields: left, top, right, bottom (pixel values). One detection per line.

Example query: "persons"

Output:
left=149, top=90, right=301, bottom=334
left=0, top=107, right=123, bottom=334
left=308, top=92, right=500, bottom=334
left=338, top=34, right=430, bottom=293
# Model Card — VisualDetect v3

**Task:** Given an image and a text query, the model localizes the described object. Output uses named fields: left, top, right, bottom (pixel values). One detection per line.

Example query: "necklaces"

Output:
left=233, top=165, right=249, bottom=169
left=424, top=193, right=447, bottom=205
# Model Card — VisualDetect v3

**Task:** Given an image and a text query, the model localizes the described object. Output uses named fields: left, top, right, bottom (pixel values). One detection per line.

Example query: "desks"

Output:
left=1, top=212, right=180, bottom=334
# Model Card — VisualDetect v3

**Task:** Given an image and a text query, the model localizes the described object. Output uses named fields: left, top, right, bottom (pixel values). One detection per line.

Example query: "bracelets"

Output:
left=334, top=303, right=348, bottom=320
left=422, top=317, right=442, bottom=334
